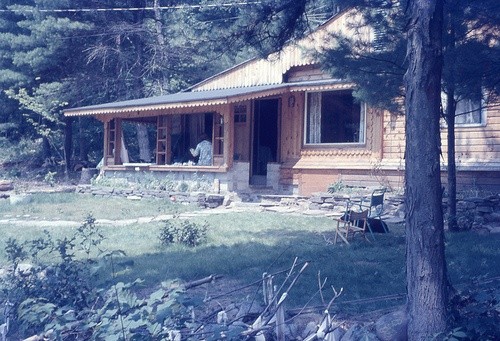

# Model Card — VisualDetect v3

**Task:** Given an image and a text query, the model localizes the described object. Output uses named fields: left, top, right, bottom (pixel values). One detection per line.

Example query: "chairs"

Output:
left=357, top=188, right=390, bottom=241
left=332, top=201, right=371, bottom=249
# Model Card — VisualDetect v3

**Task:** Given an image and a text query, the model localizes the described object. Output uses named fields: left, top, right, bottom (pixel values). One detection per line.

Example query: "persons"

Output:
left=189, top=132, right=219, bottom=166
